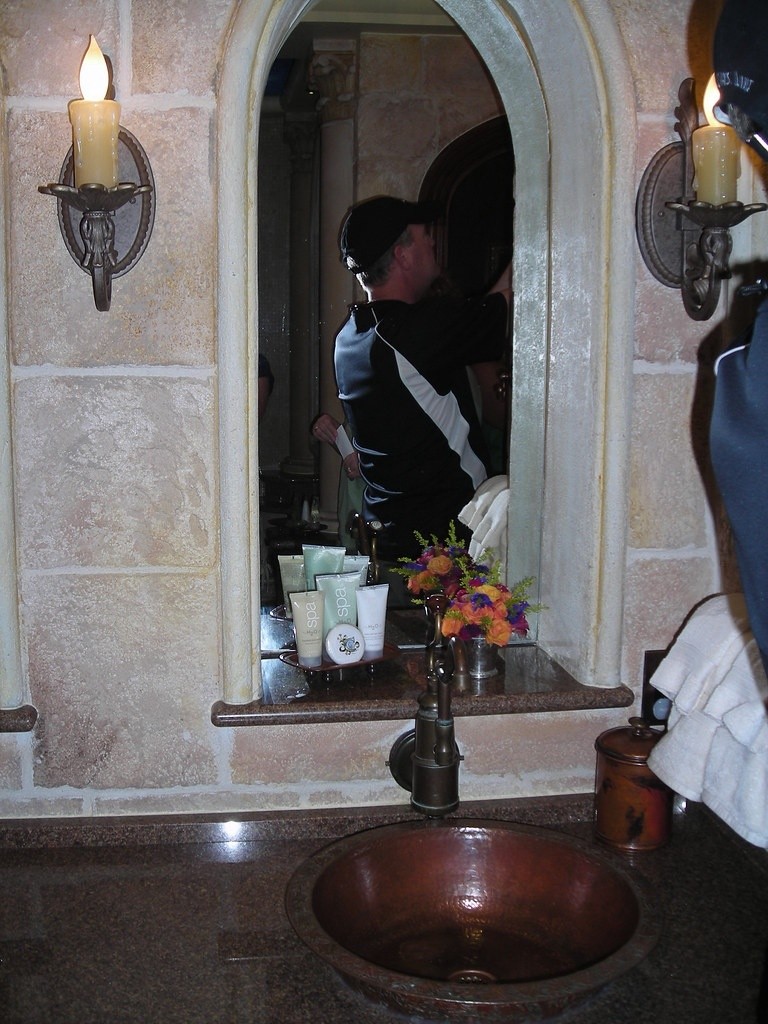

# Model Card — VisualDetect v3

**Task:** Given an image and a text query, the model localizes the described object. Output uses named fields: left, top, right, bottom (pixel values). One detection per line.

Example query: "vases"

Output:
left=422, top=592, right=451, bottom=645
left=467, top=633, right=498, bottom=679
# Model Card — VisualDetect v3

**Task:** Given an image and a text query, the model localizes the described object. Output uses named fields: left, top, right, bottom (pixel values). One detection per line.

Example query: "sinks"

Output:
left=283, top=829, right=666, bottom=1005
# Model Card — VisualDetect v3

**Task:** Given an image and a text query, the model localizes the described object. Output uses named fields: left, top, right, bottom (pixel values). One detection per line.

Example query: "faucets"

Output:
left=389, top=657, right=464, bottom=816
left=424, top=593, right=451, bottom=647
left=347, top=512, right=380, bottom=584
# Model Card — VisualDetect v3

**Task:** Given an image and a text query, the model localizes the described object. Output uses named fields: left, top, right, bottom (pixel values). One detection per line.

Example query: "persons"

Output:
left=333, top=195, right=513, bottom=564
left=708, top=1, right=768, bottom=677
left=258, top=352, right=270, bottom=421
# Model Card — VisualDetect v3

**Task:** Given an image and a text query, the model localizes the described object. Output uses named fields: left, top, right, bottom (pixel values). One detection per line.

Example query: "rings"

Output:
left=314, top=426, right=318, bottom=430
left=348, top=467, right=351, bottom=473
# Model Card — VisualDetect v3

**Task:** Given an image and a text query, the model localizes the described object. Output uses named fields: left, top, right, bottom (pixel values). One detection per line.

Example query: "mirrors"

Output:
left=257, top=0, right=541, bottom=647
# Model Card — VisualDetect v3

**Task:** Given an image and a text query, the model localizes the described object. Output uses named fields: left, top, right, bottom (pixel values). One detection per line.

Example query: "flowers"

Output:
left=389, top=518, right=496, bottom=613
left=439, top=558, right=549, bottom=648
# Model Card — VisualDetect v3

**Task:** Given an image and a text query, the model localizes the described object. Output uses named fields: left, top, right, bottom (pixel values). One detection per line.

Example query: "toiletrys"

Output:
left=277, top=544, right=371, bottom=620
left=287, top=571, right=389, bottom=668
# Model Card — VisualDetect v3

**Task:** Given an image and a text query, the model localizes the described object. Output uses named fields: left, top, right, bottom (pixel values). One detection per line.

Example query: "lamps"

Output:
left=634, top=74, right=768, bottom=321
left=37, top=34, right=156, bottom=313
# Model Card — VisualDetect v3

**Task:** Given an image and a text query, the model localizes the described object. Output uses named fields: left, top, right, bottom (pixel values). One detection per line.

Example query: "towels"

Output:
left=457, top=474, right=509, bottom=582
left=644, top=592, right=767, bottom=848
